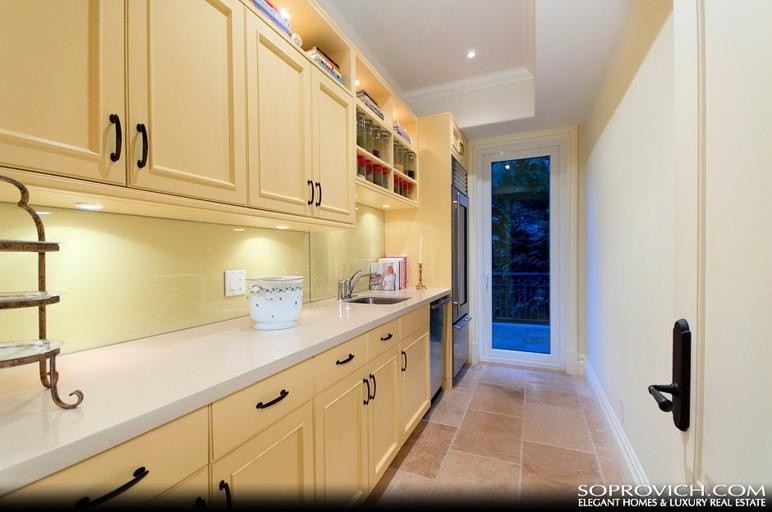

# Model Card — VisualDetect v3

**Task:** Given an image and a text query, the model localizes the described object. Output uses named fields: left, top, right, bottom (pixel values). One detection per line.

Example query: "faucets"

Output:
left=344, top=273, right=382, bottom=298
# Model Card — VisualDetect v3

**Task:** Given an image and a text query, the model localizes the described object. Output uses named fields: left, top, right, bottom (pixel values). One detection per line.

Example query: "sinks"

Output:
left=345, top=296, right=412, bottom=305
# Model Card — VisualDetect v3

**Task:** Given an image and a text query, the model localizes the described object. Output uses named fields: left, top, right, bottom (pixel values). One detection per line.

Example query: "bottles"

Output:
left=357, top=111, right=415, bottom=200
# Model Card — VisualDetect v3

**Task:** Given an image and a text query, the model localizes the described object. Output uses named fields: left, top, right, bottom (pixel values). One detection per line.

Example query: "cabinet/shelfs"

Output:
left=356, top=50, right=421, bottom=212
left=246, top=0, right=357, bottom=232
left=400, top=304, right=431, bottom=444
left=1, top=0, right=247, bottom=225
left=1, top=358, right=314, bottom=504
left=315, top=319, right=401, bottom=503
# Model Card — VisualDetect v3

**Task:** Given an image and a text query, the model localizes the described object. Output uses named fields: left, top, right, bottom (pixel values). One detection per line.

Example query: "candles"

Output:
left=418, top=234, right=423, bottom=263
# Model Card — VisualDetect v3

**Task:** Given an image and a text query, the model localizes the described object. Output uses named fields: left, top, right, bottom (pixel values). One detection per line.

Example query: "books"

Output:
left=356, top=88, right=385, bottom=120
left=251, top=0, right=293, bottom=37
left=369, top=257, right=407, bottom=291
left=393, top=119, right=411, bottom=142
left=306, top=45, right=345, bottom=85
